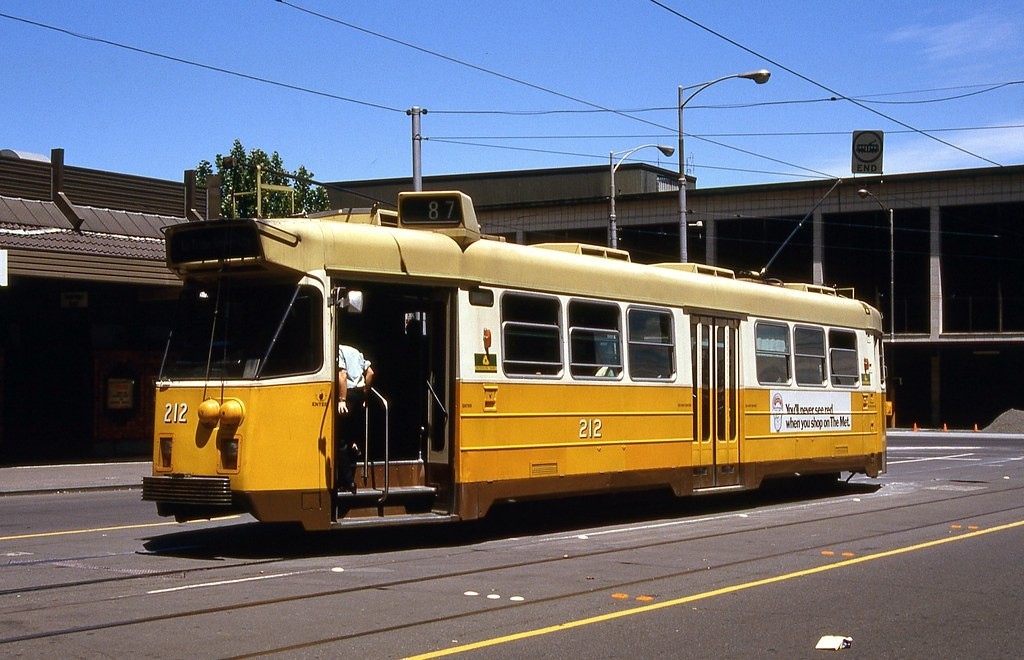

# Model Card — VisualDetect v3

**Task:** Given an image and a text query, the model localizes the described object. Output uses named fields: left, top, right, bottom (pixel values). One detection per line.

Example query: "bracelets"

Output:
left=339, top=398, right=346, bottom=402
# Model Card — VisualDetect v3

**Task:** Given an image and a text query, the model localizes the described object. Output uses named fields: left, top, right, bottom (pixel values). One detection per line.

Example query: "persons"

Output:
left=407, top=319, right=421, bottom=335
left=338, top=345, right=375, bottom=493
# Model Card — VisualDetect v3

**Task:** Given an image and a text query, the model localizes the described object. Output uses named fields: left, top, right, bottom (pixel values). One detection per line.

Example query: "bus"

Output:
left=141, top=190, right=897, bottom=535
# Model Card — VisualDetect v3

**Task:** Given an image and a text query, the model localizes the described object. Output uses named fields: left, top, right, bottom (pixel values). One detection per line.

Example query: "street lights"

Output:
left=609, top=144, right=676, bottom=250
left=678, top=69, right=771, bottom=264
left=857, top=188, right=895, bottom=342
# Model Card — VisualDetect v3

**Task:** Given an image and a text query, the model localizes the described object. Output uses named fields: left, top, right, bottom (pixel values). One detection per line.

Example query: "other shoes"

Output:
left=349, top=482, right=356, bottom=490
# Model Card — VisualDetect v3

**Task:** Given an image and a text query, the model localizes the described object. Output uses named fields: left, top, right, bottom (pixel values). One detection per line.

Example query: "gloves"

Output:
left=338, top=397, right=348, bottom=414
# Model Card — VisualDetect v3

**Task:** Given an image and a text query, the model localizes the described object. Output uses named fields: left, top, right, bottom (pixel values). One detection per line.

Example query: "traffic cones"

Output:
left=942, top=423, right=948, bottom=432
left=973, top=423, right=979, bottom=433
left=912, top=422, right=919, bottom=431
